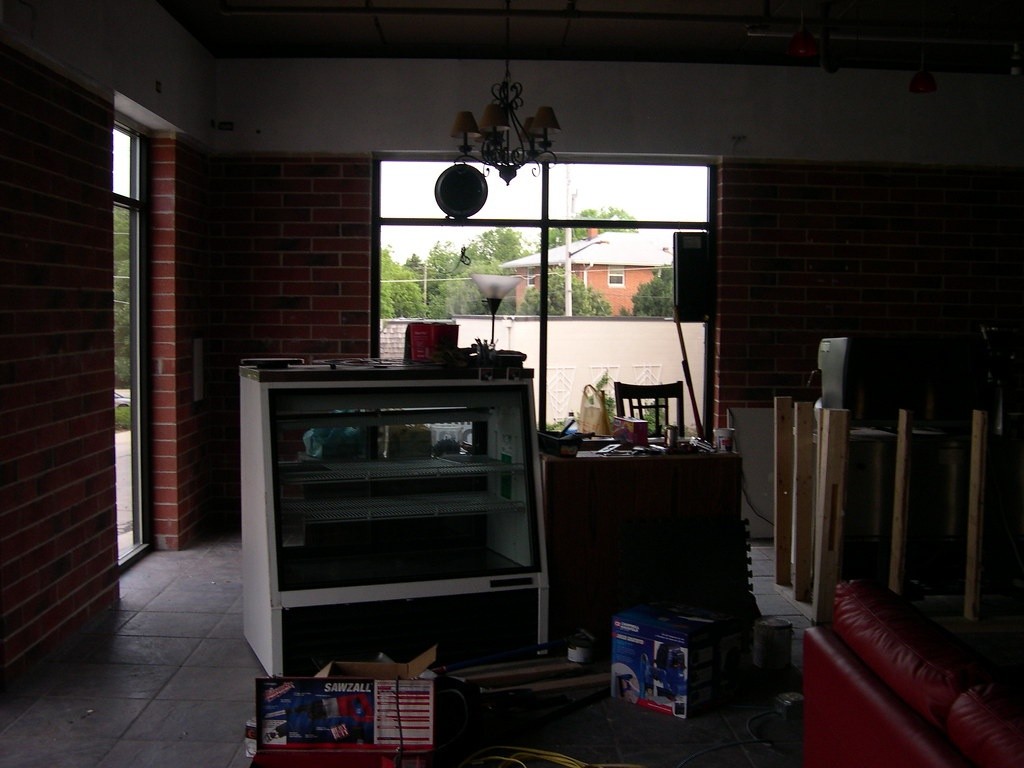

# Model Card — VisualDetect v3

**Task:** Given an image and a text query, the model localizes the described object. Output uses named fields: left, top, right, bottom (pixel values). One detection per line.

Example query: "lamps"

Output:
left=450, top=1, right=561, bottom=184
left=788, top=1, right=816, bottom=56
left=908, top=1, right=937, bottom=95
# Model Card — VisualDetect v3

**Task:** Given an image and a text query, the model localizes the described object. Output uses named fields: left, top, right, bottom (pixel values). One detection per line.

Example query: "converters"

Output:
left=773, top=691, right=803, bottom=721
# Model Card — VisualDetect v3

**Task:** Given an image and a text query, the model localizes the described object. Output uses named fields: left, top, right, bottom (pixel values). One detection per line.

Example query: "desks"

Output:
left=538, top=432, right=745, bottom=648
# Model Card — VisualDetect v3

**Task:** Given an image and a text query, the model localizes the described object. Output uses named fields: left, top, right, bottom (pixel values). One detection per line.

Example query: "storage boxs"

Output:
left=610, top=600, right=735, bottom=720
left=537, top=430, right=581, bottom=458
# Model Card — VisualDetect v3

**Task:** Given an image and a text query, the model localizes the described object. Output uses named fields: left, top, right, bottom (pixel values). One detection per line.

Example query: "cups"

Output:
left=716, top=427, right=736, bottom=453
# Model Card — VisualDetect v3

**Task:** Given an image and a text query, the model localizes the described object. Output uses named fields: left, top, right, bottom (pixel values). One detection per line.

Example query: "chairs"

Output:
left=615, top=380, right=685, bottom=439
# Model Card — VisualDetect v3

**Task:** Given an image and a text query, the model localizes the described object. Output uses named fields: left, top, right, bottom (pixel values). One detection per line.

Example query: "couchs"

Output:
left=804, top=579, right=1024, bottom=768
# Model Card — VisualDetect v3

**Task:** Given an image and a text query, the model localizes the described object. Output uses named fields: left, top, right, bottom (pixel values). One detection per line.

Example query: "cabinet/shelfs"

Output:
left=238, top=360, right=555, bottom=677
